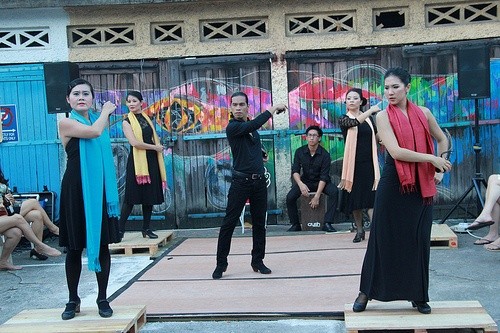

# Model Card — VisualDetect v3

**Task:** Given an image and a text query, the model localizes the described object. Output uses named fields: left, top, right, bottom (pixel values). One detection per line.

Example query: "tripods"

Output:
left=439, top=99, right=487, bottom=225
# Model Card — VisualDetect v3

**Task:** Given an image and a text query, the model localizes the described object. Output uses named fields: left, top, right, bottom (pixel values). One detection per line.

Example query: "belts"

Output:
left=232, top=169, right=264, bottom=180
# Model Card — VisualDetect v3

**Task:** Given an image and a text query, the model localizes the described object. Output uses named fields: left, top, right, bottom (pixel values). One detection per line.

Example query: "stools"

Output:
left=301, top=192, right=327, bottom=231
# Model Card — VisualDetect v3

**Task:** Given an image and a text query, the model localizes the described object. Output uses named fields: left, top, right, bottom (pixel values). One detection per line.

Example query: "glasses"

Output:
left=307, top=133, right=319, bottom=137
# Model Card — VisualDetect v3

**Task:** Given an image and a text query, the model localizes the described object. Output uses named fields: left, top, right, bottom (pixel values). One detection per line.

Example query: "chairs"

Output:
left=239, top=167, right=271, bottom=233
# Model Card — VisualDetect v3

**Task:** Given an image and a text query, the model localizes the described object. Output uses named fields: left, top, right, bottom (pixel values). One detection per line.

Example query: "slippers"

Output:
left=473, top=238, right=494, bottom=245
left=464, top=220, right=494, bottom=230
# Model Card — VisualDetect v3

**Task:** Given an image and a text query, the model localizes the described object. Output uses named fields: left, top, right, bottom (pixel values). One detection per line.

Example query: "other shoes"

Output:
left=322, top=222, right=337, bottom=232
left=29, top=249, right=48, bottom=260
left=287, top=223, right=302, bottom=232
left=482, top=243, right=500, bottom=251
left=96, top=297, right=113, bottom=318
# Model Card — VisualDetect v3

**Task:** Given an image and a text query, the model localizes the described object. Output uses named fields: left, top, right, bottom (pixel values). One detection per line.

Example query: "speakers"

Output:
left=43, top=62, right=79, bottom=114
left=457, top=45, right=491, bottom=99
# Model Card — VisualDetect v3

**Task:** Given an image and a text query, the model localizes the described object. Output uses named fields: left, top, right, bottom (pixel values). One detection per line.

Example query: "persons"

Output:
left=59, top=78, right=120, bottom=321
left=347, top=68, right=449, bottom=315
left=0, top=167, right=59, bottom=261
left=338, top=88, right=382, bottom=243
left=212, top=92, right=288, bottom=280
left=107, top=91, right=166, bottom=243
left=287, top=126, right=338, bottom=232
left=465, top=175, right=500, bottom=251
left=0, top=184, right=61, bottom=270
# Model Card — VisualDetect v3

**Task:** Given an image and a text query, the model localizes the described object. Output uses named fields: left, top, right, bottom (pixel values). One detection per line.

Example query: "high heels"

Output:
left=353, top=230, right=365, bottom=243
left=251, top=261, right=271, bottom=274
left=352, top=292, right=369, bottom=312
left=412, top=302, right=431, bottom=314
left=212, top=265, right=228, bottom=279
left=142, top=228, right=158, bottom=238
left=62, top=298, right=80, bottom=320
